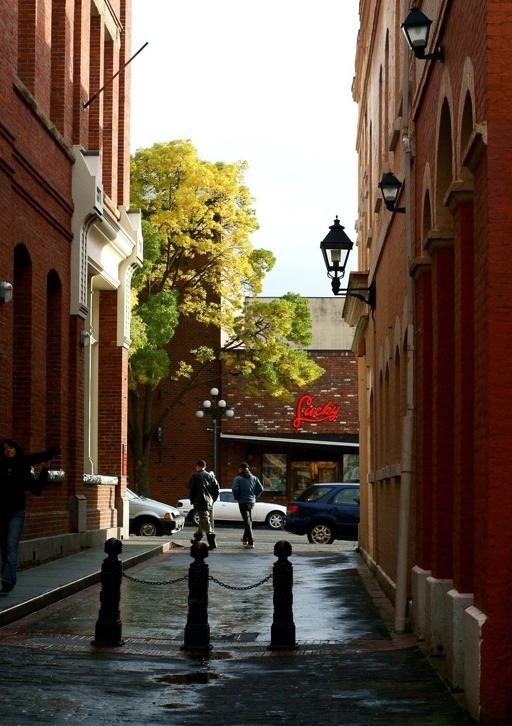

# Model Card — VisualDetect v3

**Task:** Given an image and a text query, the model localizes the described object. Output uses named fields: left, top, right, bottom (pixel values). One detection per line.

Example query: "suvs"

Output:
left=284, top=482, right=359, bottom=544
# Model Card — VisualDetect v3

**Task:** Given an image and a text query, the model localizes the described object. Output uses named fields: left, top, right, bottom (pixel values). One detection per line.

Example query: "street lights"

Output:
left=195, top=387, right=234, bottom=475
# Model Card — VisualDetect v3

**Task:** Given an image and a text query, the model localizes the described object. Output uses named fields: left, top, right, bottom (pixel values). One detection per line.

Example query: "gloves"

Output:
left=47, top=446, right=62, bottom=460
left=40, top=466, right=50, bottom=482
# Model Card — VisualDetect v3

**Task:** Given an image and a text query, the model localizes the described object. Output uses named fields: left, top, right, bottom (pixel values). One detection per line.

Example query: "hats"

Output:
left=238, top=461, right=250, bottom=469
left=195, top=459, right=207, bottom=467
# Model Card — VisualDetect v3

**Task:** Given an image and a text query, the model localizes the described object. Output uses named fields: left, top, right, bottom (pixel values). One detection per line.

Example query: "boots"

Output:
left=189, top=531, right=204, bottom=546
left=205, top=532, right=218, bottom=551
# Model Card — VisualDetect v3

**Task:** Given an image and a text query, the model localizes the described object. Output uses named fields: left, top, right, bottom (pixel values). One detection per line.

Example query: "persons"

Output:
left=232, top=462, right=264, bottom=548
left=189, top=460, right=221, bottom=550
left=2, top=437, right=61, bottom=596
left=193, top=468, right=215, bottom=538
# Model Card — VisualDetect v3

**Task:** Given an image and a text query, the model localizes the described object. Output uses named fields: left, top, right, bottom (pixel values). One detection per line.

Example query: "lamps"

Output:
left=377, top=171, right=405, bottom=214
left=400, top=4, right=444, bottom=60
left=320, top=213, right=373, bottom=307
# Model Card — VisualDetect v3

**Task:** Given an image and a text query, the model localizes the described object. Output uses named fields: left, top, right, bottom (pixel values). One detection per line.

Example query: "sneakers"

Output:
left=242, top=544, right=255, bottom=549
left=240, top=537, right=248, bottom=545
left=0, top=582, right=15, bottom=597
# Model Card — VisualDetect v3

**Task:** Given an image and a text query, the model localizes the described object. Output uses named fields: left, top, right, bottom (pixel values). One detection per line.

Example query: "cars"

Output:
left=127, top=488, right=185, bottom=537
left=176, top=489, right=288, bottom=529
